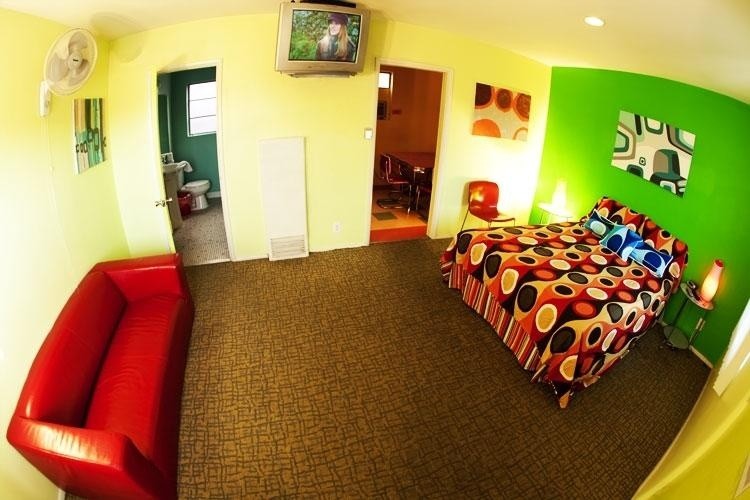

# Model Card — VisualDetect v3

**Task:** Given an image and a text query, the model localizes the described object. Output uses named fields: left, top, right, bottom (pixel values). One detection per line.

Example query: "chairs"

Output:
left=377, top=154, right=431, bottom=210
left=460, top=181, right=516, bottom=230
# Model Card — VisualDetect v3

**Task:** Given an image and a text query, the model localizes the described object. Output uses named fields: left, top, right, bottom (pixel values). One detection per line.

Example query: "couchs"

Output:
left=4, top=253, right=196, bottom=499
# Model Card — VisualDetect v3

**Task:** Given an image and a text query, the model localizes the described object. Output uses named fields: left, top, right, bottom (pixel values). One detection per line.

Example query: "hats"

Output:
left=328, top=12, right=348, bottom=25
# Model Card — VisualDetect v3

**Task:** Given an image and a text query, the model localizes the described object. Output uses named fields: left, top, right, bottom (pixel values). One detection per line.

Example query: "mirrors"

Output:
left=158, top=94, right=170, bottom=155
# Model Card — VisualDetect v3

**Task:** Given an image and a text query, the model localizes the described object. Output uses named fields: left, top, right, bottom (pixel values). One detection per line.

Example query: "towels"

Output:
left=164, top=160, right=194, bottom=173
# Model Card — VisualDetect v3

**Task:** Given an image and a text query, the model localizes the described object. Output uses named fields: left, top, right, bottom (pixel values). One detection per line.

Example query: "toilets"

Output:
left=182, top=179, right=211, bottom=210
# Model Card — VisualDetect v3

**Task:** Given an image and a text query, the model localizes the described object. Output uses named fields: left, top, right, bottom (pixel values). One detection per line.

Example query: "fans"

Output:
left=38, top=28, right=97, bottom=118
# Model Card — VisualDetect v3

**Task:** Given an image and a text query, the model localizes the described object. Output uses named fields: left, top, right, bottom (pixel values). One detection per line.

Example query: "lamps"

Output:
left=699, top=259, right=724, bottom=302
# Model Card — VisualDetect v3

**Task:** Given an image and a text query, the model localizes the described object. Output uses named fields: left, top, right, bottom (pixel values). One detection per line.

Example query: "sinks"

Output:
left=162, top=161, right=186, bottom=174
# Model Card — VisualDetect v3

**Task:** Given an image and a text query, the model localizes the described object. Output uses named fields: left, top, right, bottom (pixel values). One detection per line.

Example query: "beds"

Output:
left=437, top=196, right=690, bottom=410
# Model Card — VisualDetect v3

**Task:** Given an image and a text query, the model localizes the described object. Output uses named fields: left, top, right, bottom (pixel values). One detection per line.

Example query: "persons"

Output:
left=315, top=13, right=355, bottom=62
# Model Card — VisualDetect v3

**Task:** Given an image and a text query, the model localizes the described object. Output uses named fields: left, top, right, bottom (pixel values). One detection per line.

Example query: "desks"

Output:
left=384, top=152, right=434, bottom=212
left=658, top=283, right=715, bottom=355
left=539, top=203, right=575, bottom=223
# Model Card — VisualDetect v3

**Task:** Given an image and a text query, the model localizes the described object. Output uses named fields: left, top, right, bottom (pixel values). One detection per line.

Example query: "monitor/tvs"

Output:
left=274, top=1, right=371, bottom=75
left=377, top=101, right=387, bottom=119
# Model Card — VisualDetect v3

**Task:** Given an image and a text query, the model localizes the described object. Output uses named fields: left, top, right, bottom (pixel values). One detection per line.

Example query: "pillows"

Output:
left=582, top=208, right=674, bottom=279
